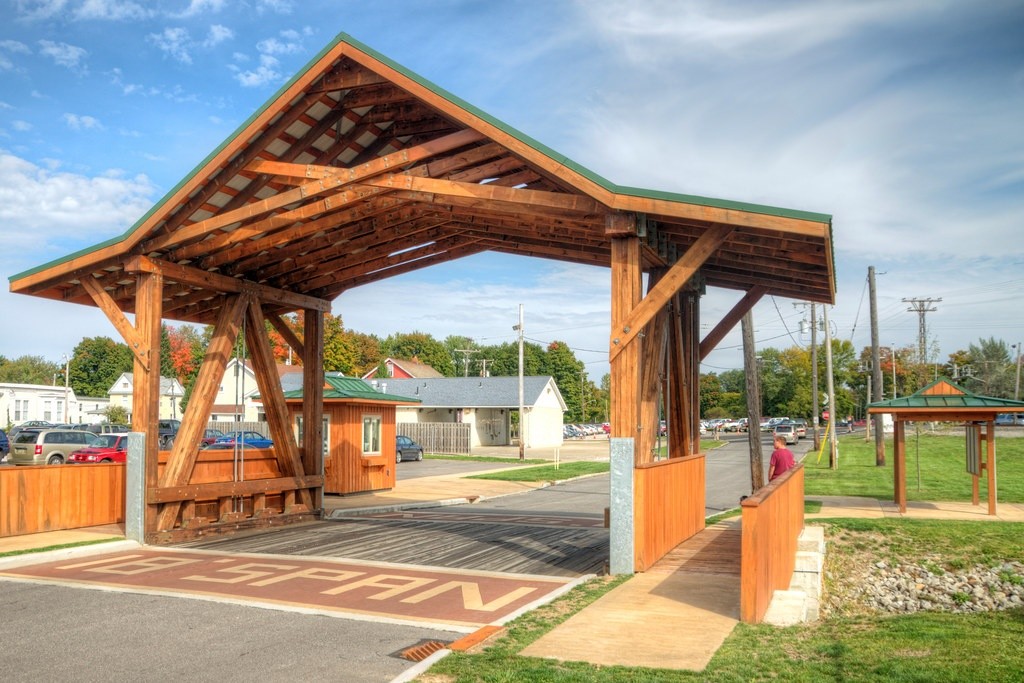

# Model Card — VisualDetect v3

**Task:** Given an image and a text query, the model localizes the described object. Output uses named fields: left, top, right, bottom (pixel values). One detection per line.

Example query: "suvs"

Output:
left=86, top=425, right=133, bottom=437
left=54, top=423, right=91, bottom=434
left=67, top=432, right=131, bottom=464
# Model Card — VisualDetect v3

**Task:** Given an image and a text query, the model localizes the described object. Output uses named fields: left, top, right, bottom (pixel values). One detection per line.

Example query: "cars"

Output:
left=217, top=431, right=275, bottom=450
left=561, top=415, right=809, bottom=444
left=201, top=442, right=259, bottom=450
left=0, top=430, right=11, bottom=466
left=200, top=428, right=225, bottom=450
left=395, top=435, right=425, bottom=464
left=157, top=418, right=182, bottom=438
left=9, top=419, right=55, bottom=436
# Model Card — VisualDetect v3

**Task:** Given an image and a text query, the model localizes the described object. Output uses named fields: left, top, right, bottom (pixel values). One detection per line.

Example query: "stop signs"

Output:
left=822, top=410, right=830, bottom=420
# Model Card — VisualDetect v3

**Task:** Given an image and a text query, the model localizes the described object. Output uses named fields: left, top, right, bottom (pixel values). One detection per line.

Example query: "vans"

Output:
left=10, top=427, right=108, bottom=467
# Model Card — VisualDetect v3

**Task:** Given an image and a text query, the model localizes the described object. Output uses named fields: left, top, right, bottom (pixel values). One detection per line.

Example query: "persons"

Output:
left=768, top=436, right=794, bottom=482
left=847, top=415, right=855, bottom=432
